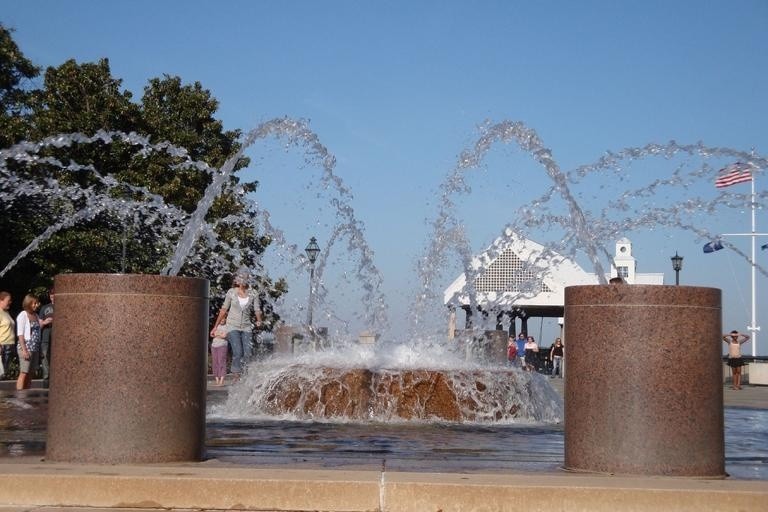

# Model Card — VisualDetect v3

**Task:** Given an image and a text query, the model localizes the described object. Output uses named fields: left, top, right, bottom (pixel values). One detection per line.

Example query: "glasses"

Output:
left=557, top=341, right=561, bottom=343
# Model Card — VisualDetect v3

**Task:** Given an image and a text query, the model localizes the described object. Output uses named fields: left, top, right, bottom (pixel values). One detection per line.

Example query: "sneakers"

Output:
left=559, top=377, right=562, bottom=378
left=551, top=376, right=555, bottom=379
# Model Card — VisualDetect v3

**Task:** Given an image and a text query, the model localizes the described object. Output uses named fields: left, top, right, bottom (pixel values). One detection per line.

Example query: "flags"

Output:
left=714, top=160, right=753, bottom=189
left=702, top=238, right=724, bottom=253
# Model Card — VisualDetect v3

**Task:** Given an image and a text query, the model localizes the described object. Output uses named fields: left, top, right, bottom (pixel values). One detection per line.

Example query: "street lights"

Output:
left=670, top=249, right=684, bottom=286
left=304, top=235, right=322, bottom=328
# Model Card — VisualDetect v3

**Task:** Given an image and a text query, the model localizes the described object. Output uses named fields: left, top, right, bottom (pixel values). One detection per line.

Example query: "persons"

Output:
left=210, top=316, right=229, bottom=384
left=721, top=330, right=750, bottom=390
left=490, top=323, right=540, bottom=373
left=549, top=338, right=564, bottom=379
left=0, top=290, right=16, bottom=381
left=38, top=288, right=55, bottom=403
left=209, top=271, right=262, bottom=385
left=13, top=292, right=54, bottom=407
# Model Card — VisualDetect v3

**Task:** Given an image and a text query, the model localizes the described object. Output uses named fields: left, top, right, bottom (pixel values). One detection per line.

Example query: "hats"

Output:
left=511, top=334, right=515, bottom=338
